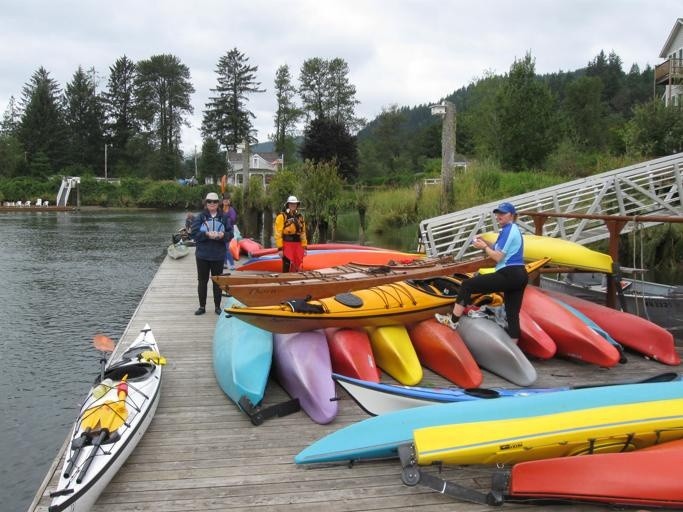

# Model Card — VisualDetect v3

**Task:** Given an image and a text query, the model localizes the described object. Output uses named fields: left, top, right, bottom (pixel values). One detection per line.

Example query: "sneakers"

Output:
left=193, top=305, right=206, bottom=316
left=222, top=264, right=227, bottom=268
left=433, top=311, right=459, bottom=331
left=213, top=307, right=222, bottom=315
left=229, top=265, right=234, bottom=270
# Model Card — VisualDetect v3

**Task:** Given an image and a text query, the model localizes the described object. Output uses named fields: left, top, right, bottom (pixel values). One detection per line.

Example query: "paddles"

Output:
left=94, top=336, right=114, bottom=384
left=64, top=401, right=129, bottom=482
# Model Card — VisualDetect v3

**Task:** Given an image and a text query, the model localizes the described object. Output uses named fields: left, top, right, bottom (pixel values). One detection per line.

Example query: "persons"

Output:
left=220, top=195, right=238, bottom=272
left=189, top=192, right=234, bottom=317
left=273, top=194, right=309, bottom=274
left=431, top=201, right=529, bottom=348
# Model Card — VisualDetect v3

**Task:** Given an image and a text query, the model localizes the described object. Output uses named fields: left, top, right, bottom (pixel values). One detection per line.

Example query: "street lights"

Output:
left=103, top=143, right=114, bottom=181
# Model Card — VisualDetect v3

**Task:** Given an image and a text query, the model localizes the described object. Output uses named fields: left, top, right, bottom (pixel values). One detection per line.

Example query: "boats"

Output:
left=476, top=230, right=616, bottom=277
left=167, top=240, right=187, bottom=260
left=46, top=320, right=161, bottom=511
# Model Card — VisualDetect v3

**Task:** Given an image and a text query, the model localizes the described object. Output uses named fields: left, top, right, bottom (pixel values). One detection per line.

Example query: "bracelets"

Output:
left=482, top=244, right=487, bottom=252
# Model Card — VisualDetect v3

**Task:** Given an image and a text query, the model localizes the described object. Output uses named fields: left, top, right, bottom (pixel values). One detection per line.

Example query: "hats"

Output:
left=285, top=195, right=300, bottom=204
left=204, top=192, right=218, bottom=201
left=491, top=200, right=516, bottom=216
